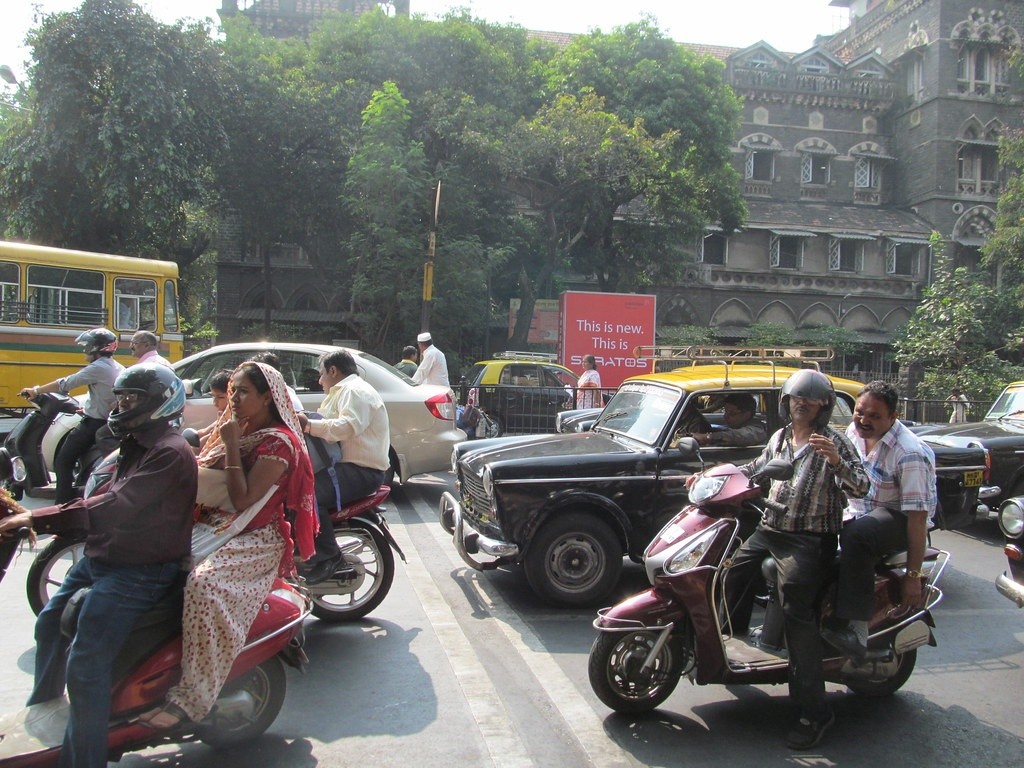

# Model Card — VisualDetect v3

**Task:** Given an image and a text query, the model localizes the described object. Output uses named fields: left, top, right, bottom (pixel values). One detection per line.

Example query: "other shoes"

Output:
left=789, top=706, right=835, bottom=749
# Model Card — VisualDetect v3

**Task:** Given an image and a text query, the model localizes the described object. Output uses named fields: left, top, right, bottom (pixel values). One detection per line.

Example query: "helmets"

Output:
left=107, top=361, right=186, bottom=438
left=779, top=368, right=838, bottom=428
left=75, top=328, right=117, bottom=354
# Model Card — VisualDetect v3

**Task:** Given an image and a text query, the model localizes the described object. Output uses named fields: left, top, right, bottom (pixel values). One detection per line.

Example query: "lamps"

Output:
left=840, top=293, right=850, bottom=316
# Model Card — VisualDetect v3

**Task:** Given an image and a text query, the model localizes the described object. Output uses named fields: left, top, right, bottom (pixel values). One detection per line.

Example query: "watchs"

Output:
left=907, top=570, right=922, bottom=578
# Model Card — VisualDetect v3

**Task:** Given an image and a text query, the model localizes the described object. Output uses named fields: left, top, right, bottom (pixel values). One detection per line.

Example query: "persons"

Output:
left=649, top=395, right=713, bottom=443
left=946, top=388, right=970, bottom=425
left=95, top=330, right=176, bottom=468
left=572, top=354, right=603, bottom=410
left=117, top=292, right=142, bottom=330
left=753, top=380, right=937, bottom=653
left=393, top=346, right=418, bottom=381
left=411, top=332, right=452, bottom=388
left=19, top=327, right=128, bottom=506
left=686, top=369, right=872, bottom=752
left=695, top=395, right=732, bottom=413
left=0, top=361, right=198, bottom=768
left=691, top=393, right=768, bottom=446
left=140, top=353, right=314, bottom=728
left=292, top=350, right=391, bottom=585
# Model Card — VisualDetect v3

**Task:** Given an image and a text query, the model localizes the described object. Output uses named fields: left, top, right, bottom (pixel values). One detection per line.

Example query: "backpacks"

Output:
left=296, top=411, right=344, bottom=513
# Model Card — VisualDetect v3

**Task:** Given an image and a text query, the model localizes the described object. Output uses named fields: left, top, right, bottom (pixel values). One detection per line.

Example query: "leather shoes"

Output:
left=823, top=628, right=867, bottom=660
left=305, top=553, right=348, bottom=585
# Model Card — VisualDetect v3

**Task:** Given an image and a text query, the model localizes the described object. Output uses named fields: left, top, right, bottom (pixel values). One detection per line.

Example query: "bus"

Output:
left=0, top=240, right=188, bottom=413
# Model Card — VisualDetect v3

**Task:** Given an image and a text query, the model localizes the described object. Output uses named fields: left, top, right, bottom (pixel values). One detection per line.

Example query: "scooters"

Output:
left=0, top=448, right=314, bottom=768
left=0, top=389, right=123, bottom=503
left=25, top=427, right=409, bottom=626
left=588, top=436, right=951, bottom=714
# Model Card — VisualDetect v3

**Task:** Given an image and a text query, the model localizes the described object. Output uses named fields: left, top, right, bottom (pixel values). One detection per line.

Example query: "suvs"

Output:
left=437, top=347, right=989, bottom=609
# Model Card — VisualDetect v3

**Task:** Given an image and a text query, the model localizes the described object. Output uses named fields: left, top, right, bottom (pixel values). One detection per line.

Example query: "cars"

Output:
left=901, top=379, right=1024, bottom=527
left=456, top=349, right=629, bottom=438
left=37, top=341, right=470, bottom=503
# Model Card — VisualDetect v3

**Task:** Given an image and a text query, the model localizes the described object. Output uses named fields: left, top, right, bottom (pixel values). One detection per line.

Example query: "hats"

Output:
left=417, top=332, right=431, bottom=342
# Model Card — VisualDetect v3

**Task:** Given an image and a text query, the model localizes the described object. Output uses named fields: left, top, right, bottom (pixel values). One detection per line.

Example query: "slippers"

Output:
left=137, top=702, right=186, bottom=731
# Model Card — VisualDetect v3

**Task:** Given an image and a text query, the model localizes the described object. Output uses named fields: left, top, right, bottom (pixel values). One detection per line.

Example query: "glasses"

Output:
left=116, top=394, right=137, bottom=402
left=723, top=410, right=745, bottom=418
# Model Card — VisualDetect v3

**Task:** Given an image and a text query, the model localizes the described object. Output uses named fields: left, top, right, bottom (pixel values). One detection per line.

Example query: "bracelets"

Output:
left=303, top=419, right=311, bottom=434
left=223, top=465, right=243, bottom=470
left=32, top=386, right=40, bottom=396
left=706, top=432, right=712, bottom=444
